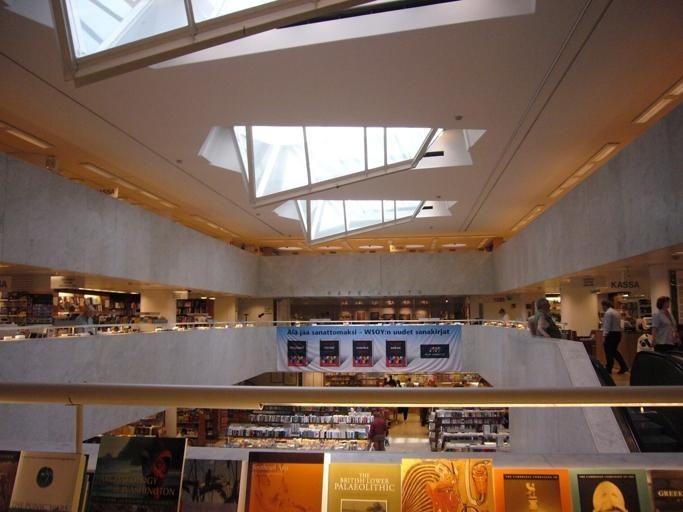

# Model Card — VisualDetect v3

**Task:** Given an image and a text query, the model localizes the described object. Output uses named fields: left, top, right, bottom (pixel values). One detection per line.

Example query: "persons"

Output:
left=497, top=296, right=677, bottom=374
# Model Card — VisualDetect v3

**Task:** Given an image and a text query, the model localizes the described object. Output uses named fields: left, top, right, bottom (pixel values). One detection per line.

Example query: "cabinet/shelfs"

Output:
left=427, top=406, right=509, bottom=454
left=113, top=406, right=389, bottom=452
left=2, top=288, right=213, bottom=341
left=323, top=371, right=480, bottom=387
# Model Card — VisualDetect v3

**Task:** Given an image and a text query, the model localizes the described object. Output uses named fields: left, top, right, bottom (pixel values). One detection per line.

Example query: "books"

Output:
left=0, top=292, right=205, bottom=337
